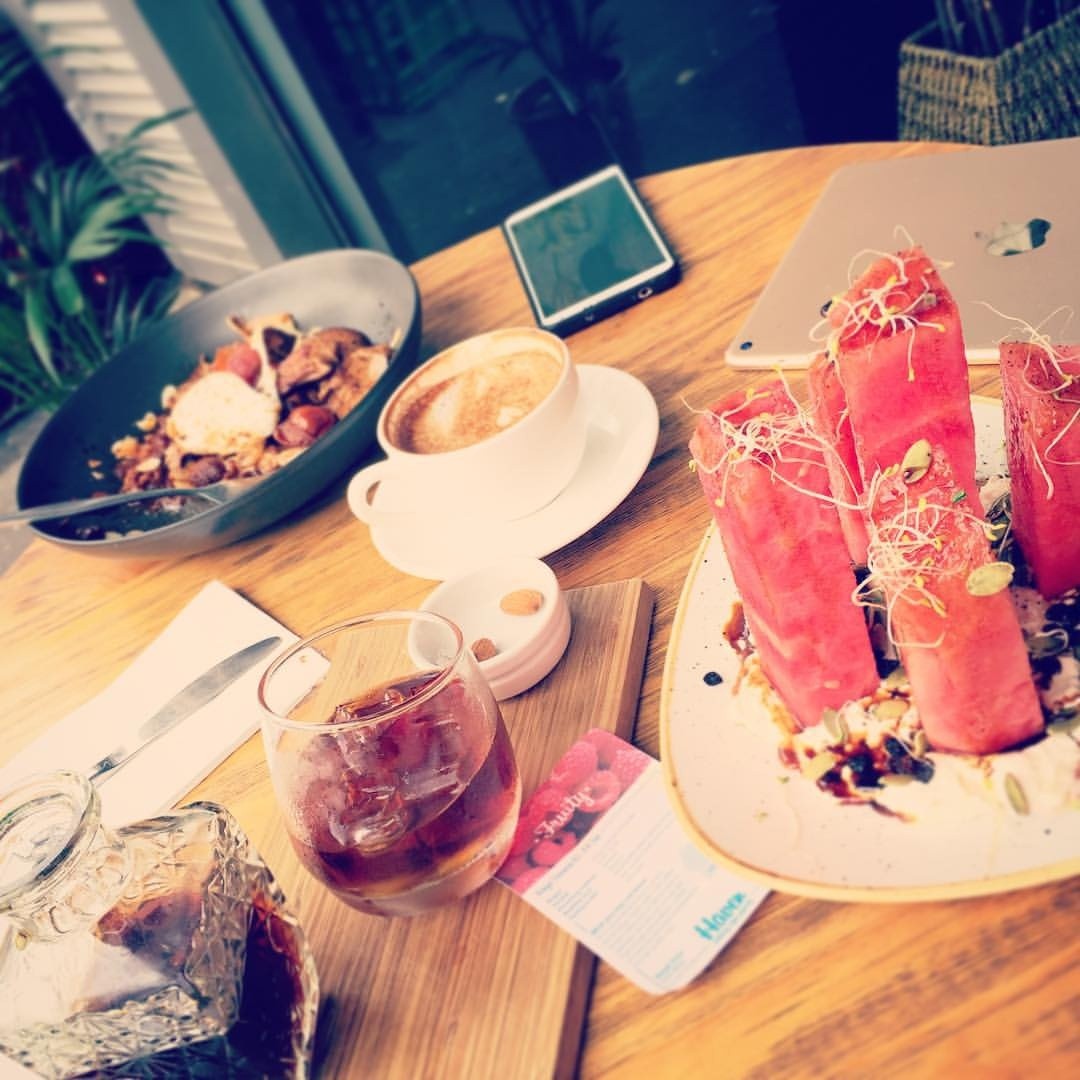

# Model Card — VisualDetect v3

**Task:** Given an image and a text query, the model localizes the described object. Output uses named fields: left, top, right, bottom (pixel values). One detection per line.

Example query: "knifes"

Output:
left=81, top=633, right=281, bottom=787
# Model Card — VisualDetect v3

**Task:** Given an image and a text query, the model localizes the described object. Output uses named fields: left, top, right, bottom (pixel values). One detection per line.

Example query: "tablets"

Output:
left=725, top=135, right=1080, bottom=371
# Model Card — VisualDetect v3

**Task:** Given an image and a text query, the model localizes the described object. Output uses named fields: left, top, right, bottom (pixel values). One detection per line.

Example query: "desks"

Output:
left=0, top=141, right=1080, bottom=1080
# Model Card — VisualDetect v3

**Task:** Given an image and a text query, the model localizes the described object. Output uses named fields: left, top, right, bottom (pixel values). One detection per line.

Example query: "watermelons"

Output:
left=691, top=245, right=1080, bottom=759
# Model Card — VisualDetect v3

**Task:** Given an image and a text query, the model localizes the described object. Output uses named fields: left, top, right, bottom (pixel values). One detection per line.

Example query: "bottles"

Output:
left=0, top=763, right=321, bottom=1079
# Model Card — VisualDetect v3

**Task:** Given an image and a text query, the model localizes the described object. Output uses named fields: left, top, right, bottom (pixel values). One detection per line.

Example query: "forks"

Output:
left=0, top=472, right=266, bottom=531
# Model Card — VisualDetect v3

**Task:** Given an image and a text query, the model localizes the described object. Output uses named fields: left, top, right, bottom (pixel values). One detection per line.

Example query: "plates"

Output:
left=368, top=361, right=659, bottom=581
left=658, top=393, right=1080, bottom=906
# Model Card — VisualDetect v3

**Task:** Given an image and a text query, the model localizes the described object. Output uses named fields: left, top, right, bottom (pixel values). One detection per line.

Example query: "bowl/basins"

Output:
left=15, top=248, right=423, bottom=562
left=406, top=553, right=572, bottom=702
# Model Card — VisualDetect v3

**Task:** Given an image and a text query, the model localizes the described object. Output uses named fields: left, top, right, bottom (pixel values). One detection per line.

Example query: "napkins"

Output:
left=0, top=577, right=328, bottom=829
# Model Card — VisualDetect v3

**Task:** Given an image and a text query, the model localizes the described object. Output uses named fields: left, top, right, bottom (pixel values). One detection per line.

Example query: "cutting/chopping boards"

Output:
left=253, top=581, right=655, bottom=1080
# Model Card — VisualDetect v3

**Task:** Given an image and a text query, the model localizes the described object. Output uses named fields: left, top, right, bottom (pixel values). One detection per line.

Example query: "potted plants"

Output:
left=453, top=0, right=643, bottom=186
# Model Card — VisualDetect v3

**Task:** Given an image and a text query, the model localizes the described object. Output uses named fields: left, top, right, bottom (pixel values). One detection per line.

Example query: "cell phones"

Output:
left=504, top=162, right=682, bottom=338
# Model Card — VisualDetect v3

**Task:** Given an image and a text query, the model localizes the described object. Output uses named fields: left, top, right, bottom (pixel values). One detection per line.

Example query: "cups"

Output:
left=255, top=609, right=523, bottom=920
left=346, top=324, right=587, bottom=527
